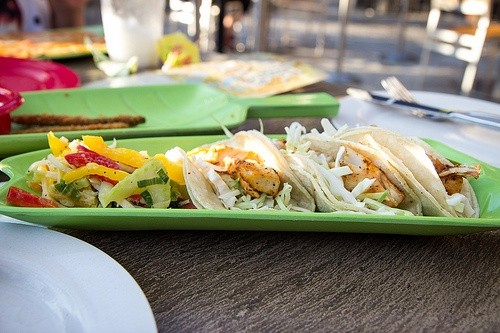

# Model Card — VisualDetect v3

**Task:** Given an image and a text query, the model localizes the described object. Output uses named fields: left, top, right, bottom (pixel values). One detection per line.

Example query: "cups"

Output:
left=100, top=1, right=165, bottom=74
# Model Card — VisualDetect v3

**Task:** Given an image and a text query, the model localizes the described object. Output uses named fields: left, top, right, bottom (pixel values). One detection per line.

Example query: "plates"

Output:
left=0, top=222, right=159, bottom=333
left=0, top=25, right=340, bottom=159
left=0, top=133, right=500, bottom=236
left=331, top=91, right=500, bottom=169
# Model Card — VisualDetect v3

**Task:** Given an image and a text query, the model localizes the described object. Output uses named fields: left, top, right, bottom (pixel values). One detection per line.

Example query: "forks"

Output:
left=380, top=75, right=446, bottom=121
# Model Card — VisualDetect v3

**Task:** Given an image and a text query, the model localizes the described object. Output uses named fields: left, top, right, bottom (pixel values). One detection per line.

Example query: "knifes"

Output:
left=346, top=88, right=500, bottom=131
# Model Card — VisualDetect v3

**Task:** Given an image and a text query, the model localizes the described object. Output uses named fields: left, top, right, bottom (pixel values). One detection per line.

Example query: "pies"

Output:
left=164, top=124, right=481, bottom=218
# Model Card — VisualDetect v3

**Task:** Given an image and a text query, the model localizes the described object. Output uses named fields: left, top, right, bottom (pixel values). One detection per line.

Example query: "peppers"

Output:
left=6, top=130, right=196, bottom=208
